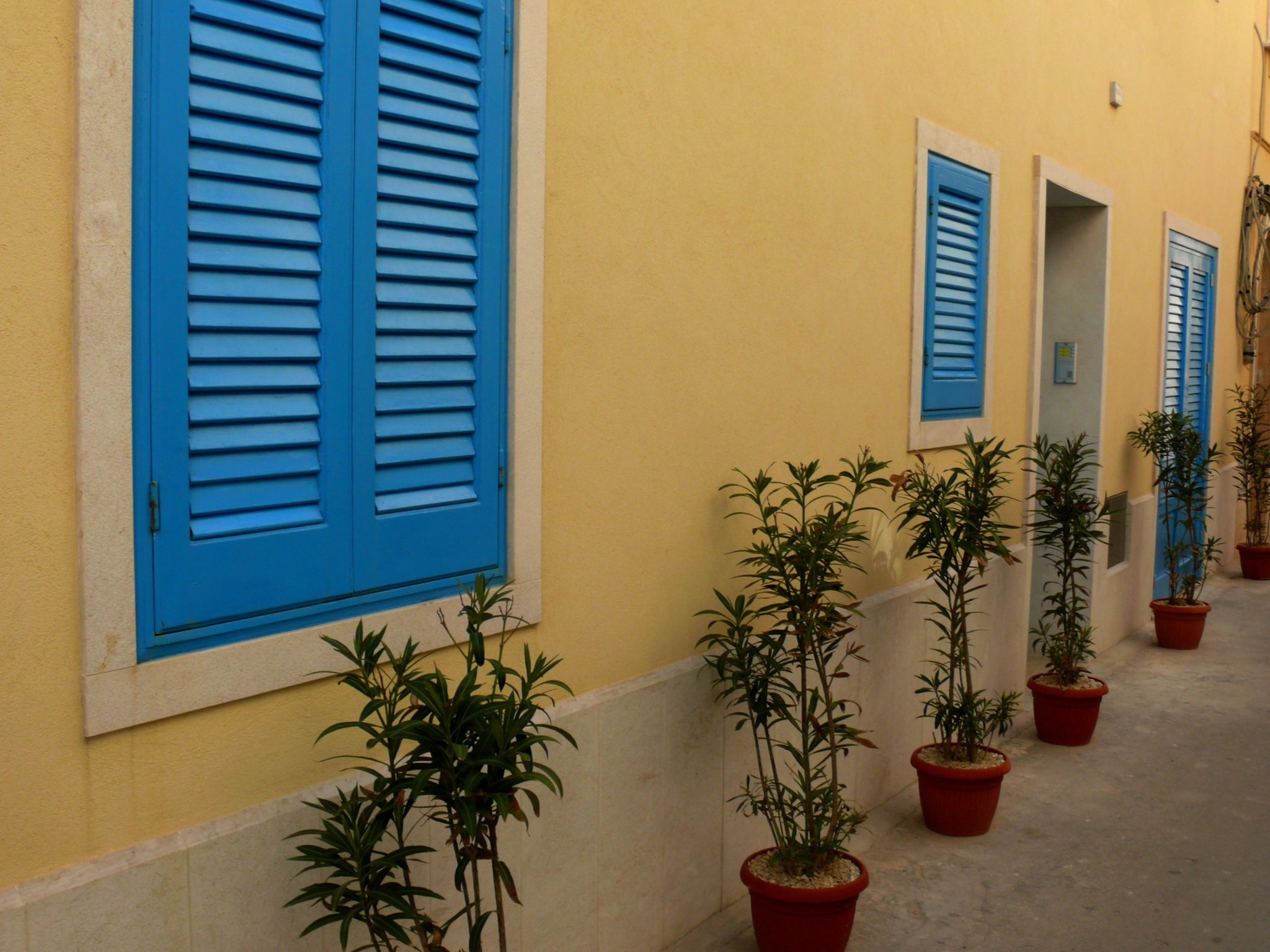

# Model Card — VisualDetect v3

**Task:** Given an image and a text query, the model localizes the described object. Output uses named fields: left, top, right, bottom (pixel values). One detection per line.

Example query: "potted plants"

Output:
left=1128, top=407, right=1230, bottom=653
left=690, top=445, right=897, bottom=950
left=883, top=428, right=1031, bottom=837
left=1010, top=434, right=1134, bottom=747
left=1219, top=377, right=1270, bottom=584
